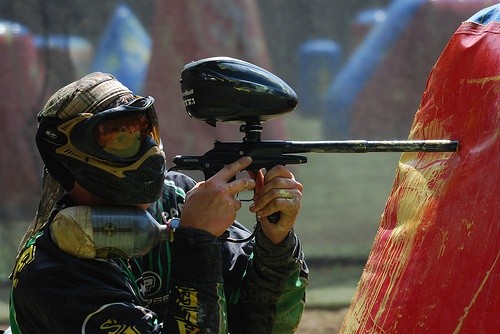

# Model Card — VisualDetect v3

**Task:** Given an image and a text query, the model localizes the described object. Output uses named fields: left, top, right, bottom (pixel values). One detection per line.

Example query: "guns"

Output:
left=166, top=55, right=459, bottom=224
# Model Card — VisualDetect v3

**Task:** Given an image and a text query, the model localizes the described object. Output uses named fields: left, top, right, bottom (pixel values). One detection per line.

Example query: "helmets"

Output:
left=35, top=97, right=165, bottom=202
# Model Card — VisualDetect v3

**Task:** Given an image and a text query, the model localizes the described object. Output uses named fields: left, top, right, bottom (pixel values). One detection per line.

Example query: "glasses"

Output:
left=91, top=105, right=161, bottom=159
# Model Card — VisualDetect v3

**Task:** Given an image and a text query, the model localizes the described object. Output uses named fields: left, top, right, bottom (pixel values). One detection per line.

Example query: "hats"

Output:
left=39, top=71, right=133, bottom=115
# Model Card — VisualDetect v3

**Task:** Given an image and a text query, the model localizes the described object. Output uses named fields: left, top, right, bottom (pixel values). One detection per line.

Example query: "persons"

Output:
left=9, top=72, right=310, bottom=334
